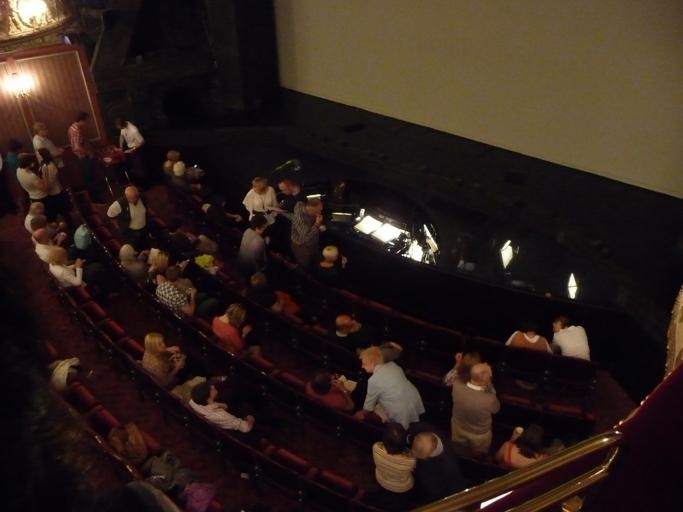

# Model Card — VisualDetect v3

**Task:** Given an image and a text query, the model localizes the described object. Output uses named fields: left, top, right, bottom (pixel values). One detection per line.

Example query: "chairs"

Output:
left=25, top=183, right=598, bottom=509
